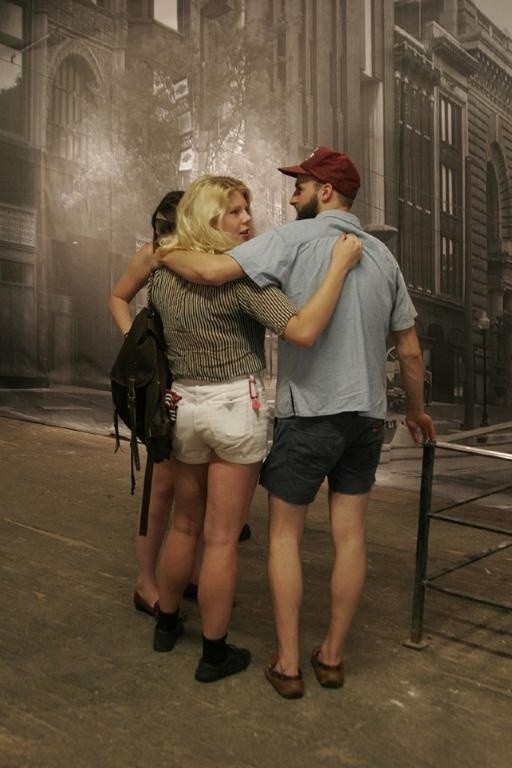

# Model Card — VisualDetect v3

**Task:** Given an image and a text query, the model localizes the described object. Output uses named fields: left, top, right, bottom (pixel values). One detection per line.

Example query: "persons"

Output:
left=147, top=144, right=436, bottom=701
left=148, top=174, right=364, bottom=684
left=108, top=190, right=199, bottom=622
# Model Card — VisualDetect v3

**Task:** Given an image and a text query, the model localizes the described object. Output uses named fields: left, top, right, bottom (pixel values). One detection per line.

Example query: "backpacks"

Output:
left=109, top=300, right=181, bottom=464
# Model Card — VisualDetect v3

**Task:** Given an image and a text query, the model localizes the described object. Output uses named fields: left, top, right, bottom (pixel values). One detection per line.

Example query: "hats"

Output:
left=277, top=146, right=360, bottom=200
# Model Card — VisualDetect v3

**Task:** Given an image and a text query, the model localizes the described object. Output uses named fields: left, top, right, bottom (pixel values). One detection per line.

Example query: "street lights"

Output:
left=474, top=306, right=498, bottom=445
left=196, top=0, right=234, bottom=175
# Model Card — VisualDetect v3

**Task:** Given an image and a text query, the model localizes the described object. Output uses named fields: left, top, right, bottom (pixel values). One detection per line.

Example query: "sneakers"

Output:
left=195, top=644, right=251, bottom=682
left=265, top=654, right=304, bottom=698
left=133, top=589, right=186, bottom=622
left=310, top=645, right=344, bottom=688
left=153, top=619, right=184, bottom=652
left=183, top=583, right=198, bottom=602
